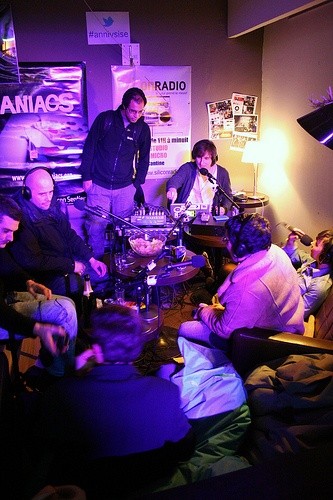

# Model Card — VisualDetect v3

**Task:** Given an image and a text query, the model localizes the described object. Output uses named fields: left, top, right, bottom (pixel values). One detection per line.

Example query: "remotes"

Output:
left=201, top=213, right=210, bottom=222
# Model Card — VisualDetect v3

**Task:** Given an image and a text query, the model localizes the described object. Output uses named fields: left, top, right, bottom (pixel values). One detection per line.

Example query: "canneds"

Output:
left=176, top=246, right=186, bottom=262
left=231, top=205, right=240, bottom=217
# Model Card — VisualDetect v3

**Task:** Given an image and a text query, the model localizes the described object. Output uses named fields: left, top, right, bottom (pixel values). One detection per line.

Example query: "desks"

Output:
left=233, top=191, right=268, bottom=212
left=116, top=247, right=200, bottom=336
left=118, top=225, right=179, bottom=242
left=184, top=224, right=227, bottom=264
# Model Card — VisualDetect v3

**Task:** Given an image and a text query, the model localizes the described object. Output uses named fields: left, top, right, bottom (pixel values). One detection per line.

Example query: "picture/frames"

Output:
left=0, top=61, right=90, bottom=204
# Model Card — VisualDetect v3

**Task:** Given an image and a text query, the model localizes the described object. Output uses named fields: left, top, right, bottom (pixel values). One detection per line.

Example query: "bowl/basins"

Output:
left=128, top=233, right=167, bottom=256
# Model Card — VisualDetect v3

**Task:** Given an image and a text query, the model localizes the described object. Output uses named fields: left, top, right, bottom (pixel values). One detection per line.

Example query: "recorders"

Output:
left=170, top=203, right=210, bottom=223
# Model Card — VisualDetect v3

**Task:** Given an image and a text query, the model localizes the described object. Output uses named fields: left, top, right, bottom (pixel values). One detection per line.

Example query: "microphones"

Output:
left=284, top=223, right=313, bottom=246
left=94, top=286, right=119, bottom=297
left=164, top=244, right=186, bottom=260
left=57, top=333, right=70, bottom=350
left=73, top=199, right=112, bottom=221
left=199, top=168, right=222, bottom=187
left=164, top=255, right=207, bottom=272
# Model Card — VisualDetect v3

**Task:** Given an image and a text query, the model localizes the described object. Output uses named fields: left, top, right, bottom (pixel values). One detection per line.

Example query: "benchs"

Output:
left=230, top=283, right=333, bottom=371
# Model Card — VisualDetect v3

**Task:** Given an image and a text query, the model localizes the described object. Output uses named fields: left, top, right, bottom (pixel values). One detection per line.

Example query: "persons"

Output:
left=0, top=194, right=78, bottom=398
left=65, top=303, right=196, bottom=496
left=166, top=140, right=234, bottom=217
left=178, top=213, right=333, bottom=352
left=80, top=88, right=151, bottom=259
left=9, top=168, right=110, bottom=323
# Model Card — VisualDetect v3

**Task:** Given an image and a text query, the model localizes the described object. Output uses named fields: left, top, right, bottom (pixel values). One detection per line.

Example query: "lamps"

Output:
left=297, top=103, right=333, bottom=150
left=241, top=141, right=265, bottom=200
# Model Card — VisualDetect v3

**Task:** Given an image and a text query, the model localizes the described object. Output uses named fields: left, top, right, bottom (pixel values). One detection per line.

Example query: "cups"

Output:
left=114, top=253, right=123, bottom=266
left=115, top=288, right=125, bottom=302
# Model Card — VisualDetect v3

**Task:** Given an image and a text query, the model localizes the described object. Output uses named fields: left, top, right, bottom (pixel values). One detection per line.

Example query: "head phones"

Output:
left=21, top=166, right=59, bottom=201
left=319, top=244, right=333, bottom=264
left=192, top=141, right=218, bottom=161
left=232, top=214, right=254, bottom=258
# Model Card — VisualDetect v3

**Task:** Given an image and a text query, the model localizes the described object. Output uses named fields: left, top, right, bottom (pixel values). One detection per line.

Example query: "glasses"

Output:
left=127, top=108, right=143, bottom=115
left=223, top=237, right=229, bottom=242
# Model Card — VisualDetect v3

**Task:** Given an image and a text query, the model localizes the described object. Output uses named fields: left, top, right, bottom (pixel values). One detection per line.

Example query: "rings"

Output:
left=171, top=192, right=175, bottom=197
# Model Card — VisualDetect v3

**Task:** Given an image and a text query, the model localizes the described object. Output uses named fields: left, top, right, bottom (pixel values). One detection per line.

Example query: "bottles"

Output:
left=82, top=274, right=96, bottom=326
